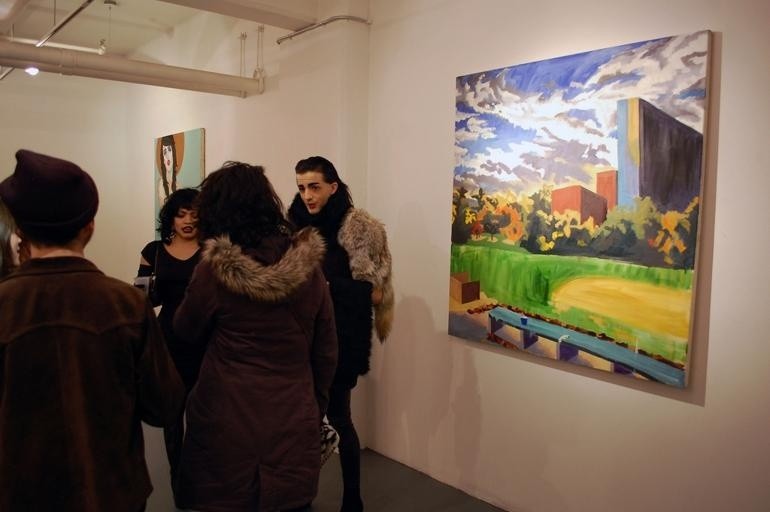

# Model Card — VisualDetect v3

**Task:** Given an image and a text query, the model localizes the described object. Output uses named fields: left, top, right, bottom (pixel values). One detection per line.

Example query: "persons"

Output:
left=172, top=161, right=339, bottom=512
left=131, top=189, right=208, bottom=509
left=157, top=136, right=182, bottom=209
left=0, top=150, right=185, bottom=512
left=0, top=202, right=22, bottom=278
left=285, top=156, right=394, bottom=512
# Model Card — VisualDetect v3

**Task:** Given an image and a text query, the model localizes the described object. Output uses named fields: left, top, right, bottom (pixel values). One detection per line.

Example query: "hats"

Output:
left=1, top=148, right=98, bottom=247
